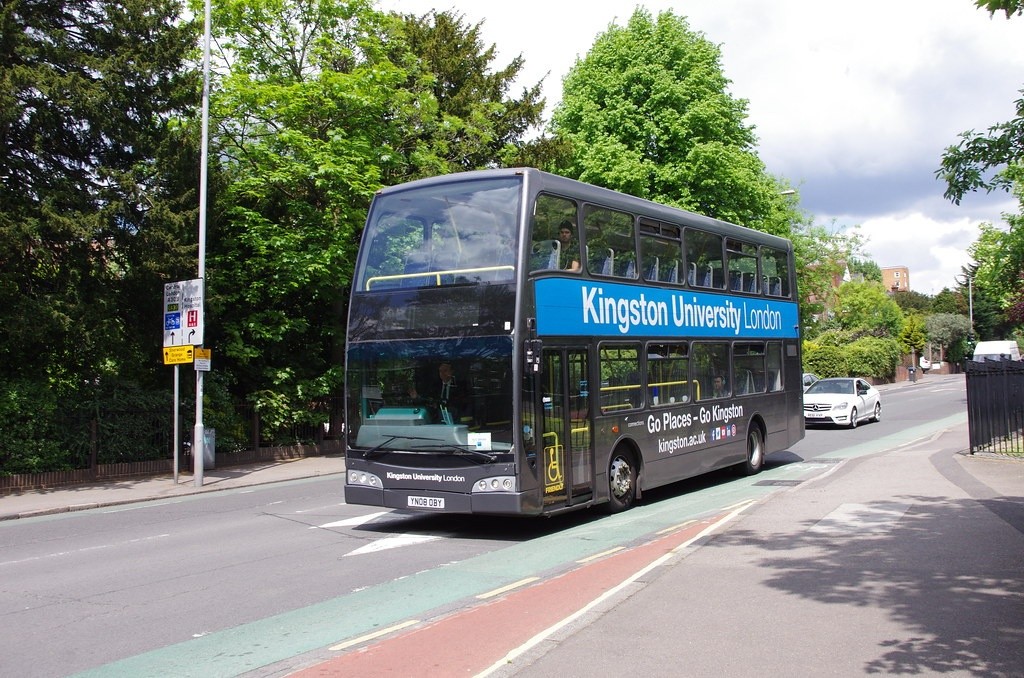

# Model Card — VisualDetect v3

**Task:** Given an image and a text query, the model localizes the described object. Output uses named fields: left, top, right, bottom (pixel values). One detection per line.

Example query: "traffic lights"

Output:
left=967, top=336, right=971, bottom=345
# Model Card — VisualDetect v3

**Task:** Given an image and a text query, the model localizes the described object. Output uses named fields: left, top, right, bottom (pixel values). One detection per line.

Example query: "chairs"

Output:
left=735, top=369, right=775, bottom=395
left=399, top=241, right=782, bottom=296
left=577, top=368, right=777, bottom=421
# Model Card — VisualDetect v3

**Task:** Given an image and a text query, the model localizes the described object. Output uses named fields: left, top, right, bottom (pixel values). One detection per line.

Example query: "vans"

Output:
left=972, top=341, right=1021, bottom=362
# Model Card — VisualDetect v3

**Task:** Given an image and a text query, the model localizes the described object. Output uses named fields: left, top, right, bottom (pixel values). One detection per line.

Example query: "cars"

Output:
left=803, top=378, right=881, bottom=429
left=802, top=373, right=818, bottom=393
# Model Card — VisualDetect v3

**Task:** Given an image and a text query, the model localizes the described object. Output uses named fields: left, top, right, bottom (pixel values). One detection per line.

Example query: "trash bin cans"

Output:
left=909, top=367, right=916, bottom=381
left=190, top=427, right=215, bottom=470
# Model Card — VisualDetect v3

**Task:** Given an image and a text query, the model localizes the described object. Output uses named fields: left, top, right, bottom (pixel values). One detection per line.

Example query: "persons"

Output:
left=713, top=375, right=729, bottom=397
left=553, top=220, right=580, bottom=271
left=406, top=363, right=460, bottom=402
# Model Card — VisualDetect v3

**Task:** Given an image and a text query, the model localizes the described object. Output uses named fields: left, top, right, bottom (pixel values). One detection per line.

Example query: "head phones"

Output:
left=721, top=375, right=725, bottom=384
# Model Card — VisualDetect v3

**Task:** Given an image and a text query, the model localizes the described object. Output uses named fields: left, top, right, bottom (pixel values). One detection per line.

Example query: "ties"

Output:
left=443, top=384, right=447, bottom=398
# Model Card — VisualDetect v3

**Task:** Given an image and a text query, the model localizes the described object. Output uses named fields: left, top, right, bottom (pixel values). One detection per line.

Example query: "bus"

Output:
left=345, top=167, right=806, bottom=518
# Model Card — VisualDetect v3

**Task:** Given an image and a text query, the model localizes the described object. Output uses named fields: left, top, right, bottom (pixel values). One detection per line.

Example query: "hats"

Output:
left=559, top=220, right=573, bottom=233
